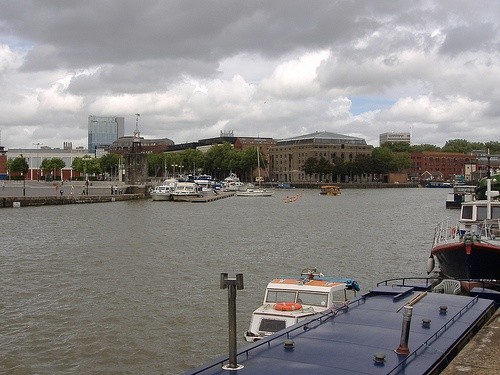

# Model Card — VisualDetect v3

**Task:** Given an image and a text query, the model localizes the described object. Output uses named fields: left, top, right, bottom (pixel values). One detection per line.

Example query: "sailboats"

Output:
left=235, top=146, right=274, bottom=197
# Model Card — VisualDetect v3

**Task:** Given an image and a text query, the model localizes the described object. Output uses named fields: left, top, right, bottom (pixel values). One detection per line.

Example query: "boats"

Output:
left=150, top=172, right=245, bottom=201
left=243, top=266, right=360, bottom=343
left=319, top=186, right=341, bottom=197
left=426, top=147, right=500, bottom=304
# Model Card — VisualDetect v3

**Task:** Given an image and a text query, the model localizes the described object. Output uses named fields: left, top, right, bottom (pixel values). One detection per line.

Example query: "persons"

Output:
left=111, top=185, right=114, bottom=195
left=60, top=187, right=64, bottom=196
left=71, top=185, right=75, bottom=195
left=82, top=186, right=86, bottom=195
left=115, top=185, right=118, bottom=194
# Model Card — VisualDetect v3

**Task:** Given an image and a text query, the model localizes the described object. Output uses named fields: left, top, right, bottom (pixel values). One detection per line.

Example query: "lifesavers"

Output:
left=273, top=302, right=302, bottom=311
